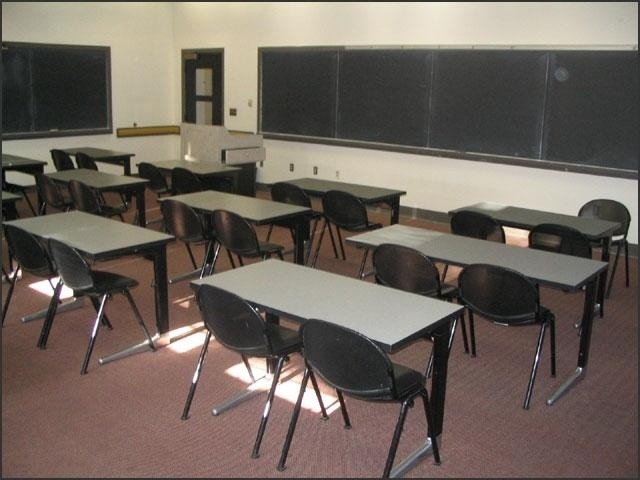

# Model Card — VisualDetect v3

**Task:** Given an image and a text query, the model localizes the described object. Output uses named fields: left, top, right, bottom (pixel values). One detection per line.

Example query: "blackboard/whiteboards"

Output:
left=1, top=41, right=113, bottom=141
left=257, top=45, right=640, bottom=179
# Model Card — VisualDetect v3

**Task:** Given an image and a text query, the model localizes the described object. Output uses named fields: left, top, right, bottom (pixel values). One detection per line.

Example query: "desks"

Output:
left=160, top=187, right=311, bottom=282
left=343, top=223, right=610, bottom=405
left=187, top=254, right=464, bottom=480
left=5, top=211, right=210, bottom=364
left=448, top=201, right=622, bottom=326
left=274, top=173, right=408, bottom=256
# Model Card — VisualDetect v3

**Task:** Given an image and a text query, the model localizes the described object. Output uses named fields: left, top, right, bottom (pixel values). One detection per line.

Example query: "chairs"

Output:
left=370, top=242, right=470, bottom=377
left=179, top=283, right=327, bottom=461
left=45, top=241, right=157, bottom=373
left=277, top=317, right=443, bottom=478
left=447, top=261, right=558, bottom=410
left=159, top=201, right=209, bottom=300
left=575, top=197, right=632, bottom=299
left=313, top=189, right=384, bottom=280
left=264, top=180, right=338, bottom=261
left=205, top=210, right=286, bottom=304
left=4, top=228, right=73, bottom=348
left=527, top=223, right=594, bottom=327
left=2, top=144, right=246, bottom=263
left=439, top=213, right=508, bottom=286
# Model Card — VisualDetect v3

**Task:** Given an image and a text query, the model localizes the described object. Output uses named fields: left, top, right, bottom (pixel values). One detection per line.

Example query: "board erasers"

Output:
left=49, top=129, right=59, bottom=132
